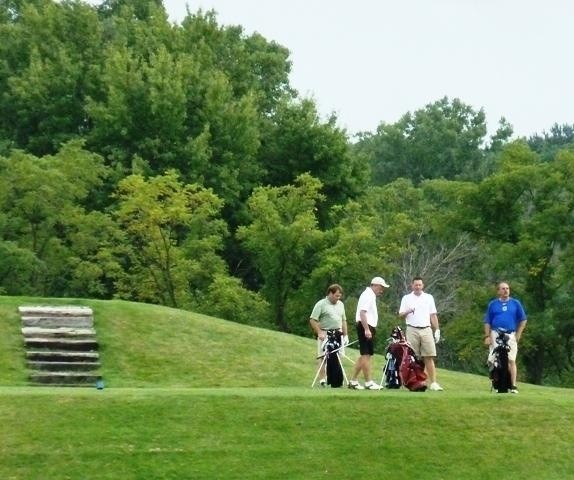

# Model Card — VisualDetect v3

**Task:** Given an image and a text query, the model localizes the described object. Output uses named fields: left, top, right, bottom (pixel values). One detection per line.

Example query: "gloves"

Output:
left=434, top=329, right=440, bottom=343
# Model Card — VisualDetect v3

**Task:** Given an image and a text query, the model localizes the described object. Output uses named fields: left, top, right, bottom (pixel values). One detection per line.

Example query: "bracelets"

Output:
left=485, top=335, right=490, bottom=338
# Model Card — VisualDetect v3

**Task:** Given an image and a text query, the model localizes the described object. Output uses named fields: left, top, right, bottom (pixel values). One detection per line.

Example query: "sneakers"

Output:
left=511, top=383, right=519, bottom=393
left=430, top=382, right=443, bottom=391
left=348, top=379, right=383, bottom=390
left=321, top=382, right=326, bottom=387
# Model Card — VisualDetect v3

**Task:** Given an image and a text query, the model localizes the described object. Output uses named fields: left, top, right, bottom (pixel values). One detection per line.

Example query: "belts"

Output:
left=407, top=324, right=430, bottom=329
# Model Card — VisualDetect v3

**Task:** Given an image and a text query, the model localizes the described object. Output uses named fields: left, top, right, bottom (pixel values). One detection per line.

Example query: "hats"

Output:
left=370, top=276, right=390, bottom=288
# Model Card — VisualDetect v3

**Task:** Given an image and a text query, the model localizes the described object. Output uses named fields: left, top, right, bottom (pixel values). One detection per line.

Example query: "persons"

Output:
left=310, top=284, right=350, bottom=385
left=349, top=277, right=390, bottom=390
left=484, top=282, right=528, bottom=394
left=399, top=277, right=443, bottom=391
